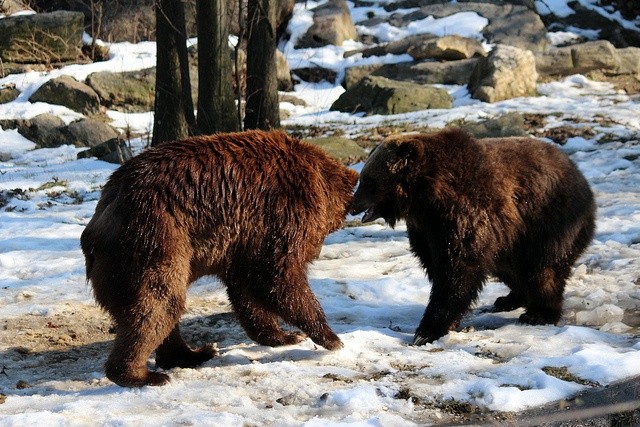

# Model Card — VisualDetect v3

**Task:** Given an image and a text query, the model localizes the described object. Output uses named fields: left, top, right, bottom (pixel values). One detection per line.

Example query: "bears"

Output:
left=349, top=129, right=597, bottom=347
left=80, top=131, right=359, bottom=389
